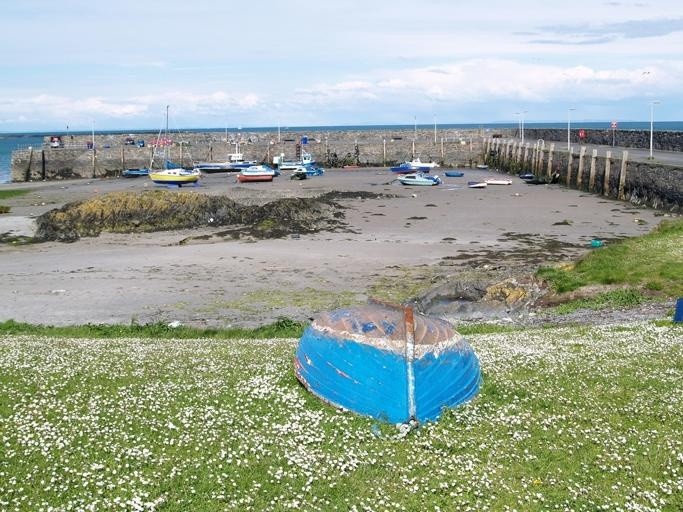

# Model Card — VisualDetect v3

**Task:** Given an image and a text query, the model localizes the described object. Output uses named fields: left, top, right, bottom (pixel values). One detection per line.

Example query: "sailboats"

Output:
left=144, top=105, right=203, bottom=188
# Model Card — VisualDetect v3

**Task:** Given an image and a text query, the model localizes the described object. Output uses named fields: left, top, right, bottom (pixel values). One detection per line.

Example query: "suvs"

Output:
left=123, top=137, right=135, bottom=145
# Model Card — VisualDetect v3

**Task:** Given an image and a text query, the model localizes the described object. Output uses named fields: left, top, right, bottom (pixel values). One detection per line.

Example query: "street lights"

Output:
left=91, top=119, right=97, bottom=149
left=567, top=108, right=575, bottom=150
left=648, top=100, right=662, bottom=155
left=515, top=110, right=530, bottom=143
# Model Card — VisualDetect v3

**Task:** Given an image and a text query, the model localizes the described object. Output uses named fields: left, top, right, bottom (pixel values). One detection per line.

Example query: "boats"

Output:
left=386, top=157, right=536, bottom=191
left=120, top=151, right=324, bottom=188
left=293, top=302, right=482, bottom=434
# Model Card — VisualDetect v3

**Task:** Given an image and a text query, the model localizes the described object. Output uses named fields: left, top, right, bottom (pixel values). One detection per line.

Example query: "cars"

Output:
left=136, top=139, right=145, bottom=147
